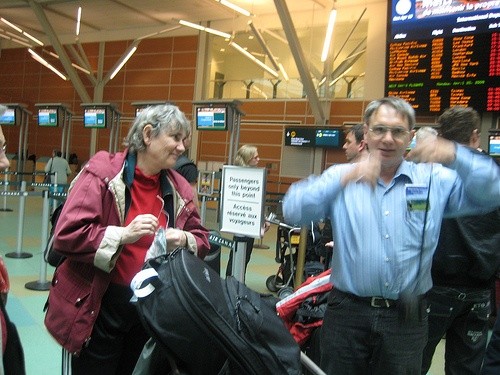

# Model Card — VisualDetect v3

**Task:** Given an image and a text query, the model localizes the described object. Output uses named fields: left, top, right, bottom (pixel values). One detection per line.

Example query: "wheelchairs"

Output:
left=265, top=223, right=324, bottom=299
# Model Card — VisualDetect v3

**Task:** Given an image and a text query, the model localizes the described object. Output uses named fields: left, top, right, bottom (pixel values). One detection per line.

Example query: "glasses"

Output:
left=367, top=126, right=411, bottom=137
left=0, top=144, right=7, bottom=153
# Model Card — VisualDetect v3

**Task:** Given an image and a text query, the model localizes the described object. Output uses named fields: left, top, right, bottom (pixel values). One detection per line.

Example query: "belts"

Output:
left=333, top=287, right=426, bottom=309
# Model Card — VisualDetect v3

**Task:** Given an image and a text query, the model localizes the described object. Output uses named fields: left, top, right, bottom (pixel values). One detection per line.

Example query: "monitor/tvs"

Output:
left=135, top=107, right=146, bottom=117
left=195, top=106, right=227, bottom=131
left=37, top=108, right=58, bottom=127
left=285, top=128, right=341, bottom=149
left=487, top=135, right=500, bottom=158
left=0, top=108, right=16, bottom=126
left=84, top=108, right=107, bottom=128
left=404, top=127, right=421, bottom=153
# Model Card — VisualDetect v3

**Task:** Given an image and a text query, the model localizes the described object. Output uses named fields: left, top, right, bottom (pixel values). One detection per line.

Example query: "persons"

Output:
left=0, top=106, right=27, bottom=375
left=45, top=152, right=71, bottom=183
left=226, top=144, right=270, bottom=282
left=283, top=99, right=499, bottom=375
left=421, top=107, right=500, bottom=375
left=46, top=104, right=210, bottom=375
left=342, top=123, right=369, bottom=163
left=173, top=122, right=199, bottom=183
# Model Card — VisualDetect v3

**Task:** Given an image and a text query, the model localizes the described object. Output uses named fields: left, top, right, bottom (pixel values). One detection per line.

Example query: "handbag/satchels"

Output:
left=2, top=321, right=26, bottom=375
left=290, top=291, right=332, bottom=349
left=43, top=174, right=51, bottom=185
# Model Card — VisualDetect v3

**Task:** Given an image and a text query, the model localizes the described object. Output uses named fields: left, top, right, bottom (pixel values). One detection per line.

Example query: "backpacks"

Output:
left=129, top=246, right=303, bottom=375
left=42, top=202, right=69, bottom=269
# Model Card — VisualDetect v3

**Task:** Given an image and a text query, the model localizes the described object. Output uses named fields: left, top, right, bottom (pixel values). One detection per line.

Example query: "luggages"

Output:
left=294, top=260, right=326, bottom=283
